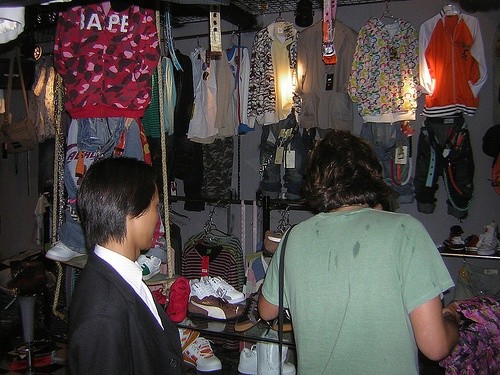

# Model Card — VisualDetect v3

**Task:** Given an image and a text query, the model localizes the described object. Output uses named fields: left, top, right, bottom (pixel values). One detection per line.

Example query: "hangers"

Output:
left=194, top=33, right=205, bottom=52
left=379, top=0, right=398, bottom=21
left=274, top=208, right=292, bottom=236
left=203, top=211, right=230, bottom=248
left=229, top=31, right=239, bottom=48
left=444, top=0, right=460, bottom=14
left=160, top=205, right=191, bottom=226
left=274, top=4, right=285, bottom=22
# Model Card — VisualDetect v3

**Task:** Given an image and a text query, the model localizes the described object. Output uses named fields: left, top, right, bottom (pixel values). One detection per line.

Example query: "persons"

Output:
left=68, top=155, right=185, bottom=375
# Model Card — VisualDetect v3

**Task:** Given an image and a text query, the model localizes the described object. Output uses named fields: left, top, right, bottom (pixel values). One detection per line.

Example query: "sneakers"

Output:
left=136, top=254, right=162, bottom=281
left=152, top=288, right=168, bottom=305
left=200, top=275, right=245, bottom=305
left=442, top=222, right=500, bottom=256
left=234, top=283, right=266, bottom=331
left=189, top=279, right=245, bottom=319
left=45, top=240, right=86, bottom=261
left=237, top=348, right=298, bottom=375
left=274, top=308, right=292, bottom=332
left=166, top=276, right=192, bottom=325
left=178, top=327, right=200, bottom=353
left=183, top=337, right=223, bottom=371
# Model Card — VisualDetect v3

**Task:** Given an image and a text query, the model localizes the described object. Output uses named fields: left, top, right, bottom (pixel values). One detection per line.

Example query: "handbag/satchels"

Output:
left=4, top=117, right=40, bottom=154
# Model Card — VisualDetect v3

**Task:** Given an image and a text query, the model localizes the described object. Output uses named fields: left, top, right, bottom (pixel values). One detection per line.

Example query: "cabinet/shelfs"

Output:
left=174, top=314, right=297, bottom=375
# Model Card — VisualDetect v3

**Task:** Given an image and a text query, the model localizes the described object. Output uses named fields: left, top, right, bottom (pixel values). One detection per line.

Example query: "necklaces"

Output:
left=257, top=131, right=463, bottom=375
left=341, top=203, right=368, bottom=208
